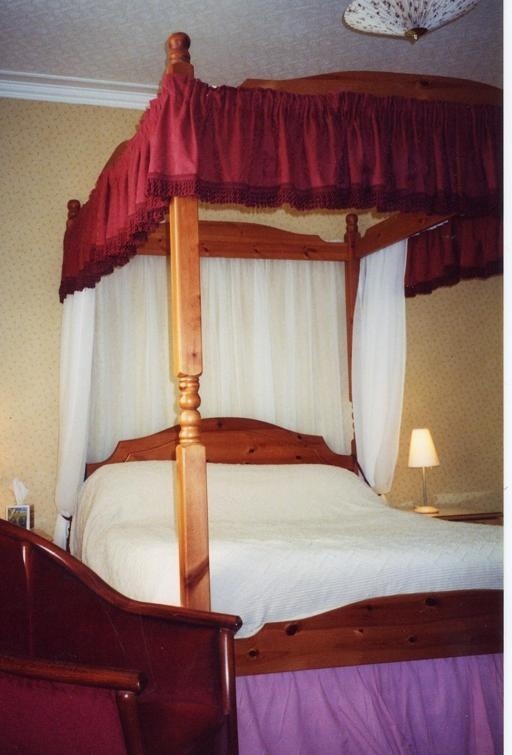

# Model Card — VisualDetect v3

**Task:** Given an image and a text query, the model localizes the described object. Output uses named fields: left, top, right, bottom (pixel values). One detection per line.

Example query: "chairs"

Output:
left=0, top=515, right=244, bottom=754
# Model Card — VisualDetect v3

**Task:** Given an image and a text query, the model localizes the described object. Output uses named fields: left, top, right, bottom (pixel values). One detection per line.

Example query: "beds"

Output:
left=51, top=27, right=503, bottom=754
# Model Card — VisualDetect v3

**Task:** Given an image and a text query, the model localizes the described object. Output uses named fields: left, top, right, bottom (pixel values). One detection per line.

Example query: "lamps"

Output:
left=337, top=1, right=481, bottom=45
left=407, top=425, right=442, bottom=517
